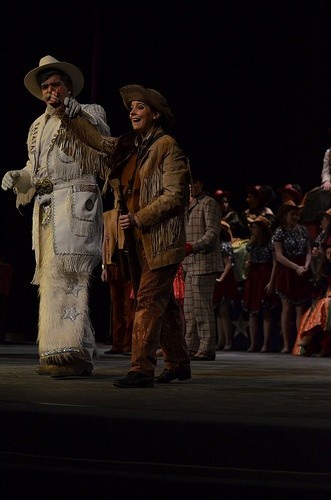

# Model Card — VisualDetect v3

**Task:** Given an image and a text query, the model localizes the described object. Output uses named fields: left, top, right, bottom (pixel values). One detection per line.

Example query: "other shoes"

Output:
left=223, top=344, right=231, bottom=350
left=261, top=345, right=269, bottom=353
left=36, top=363, right=58, bottom=374
left=154, top=367, right=191, bottom=383
left=247, top=345, right=257, bottom=352
left=281, top=348, right=290, bottom=352
left=216, top=346, right=223, bottom=350
left=112, top=369, right=153, bottom=388
left=104, top=349, right=121, bottom=354
left=190, top=352, right=215, bottom=361
left=51, top=359, right=88, bottom=377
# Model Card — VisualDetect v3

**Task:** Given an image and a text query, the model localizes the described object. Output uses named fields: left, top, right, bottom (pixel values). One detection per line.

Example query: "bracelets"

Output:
left=102, top=264, right=107, bottom=271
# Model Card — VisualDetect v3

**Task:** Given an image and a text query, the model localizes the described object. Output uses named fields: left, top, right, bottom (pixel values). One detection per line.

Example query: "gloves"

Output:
left=1, top=171, right=19, bottom=191
left=64, top=97, right=81, bottom=118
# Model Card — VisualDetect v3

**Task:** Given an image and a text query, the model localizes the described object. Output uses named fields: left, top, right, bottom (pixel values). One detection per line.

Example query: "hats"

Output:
left=214, top=190, right=231, bottom=201
left=220, top=221, right=231, bottom=231
left=277, top=200, right=305, bottom=218
left=277, top=183, right=303, bottom=201
left=248, top=184, right=265, bottom=204
left=247, top=215, right=272, bottom=234
left=24, top=55, right=84, bottom=102
left=120, top=85, right=173, bottom=131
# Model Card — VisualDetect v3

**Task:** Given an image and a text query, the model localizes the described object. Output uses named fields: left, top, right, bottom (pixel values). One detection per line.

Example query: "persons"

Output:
left=0, top=55, right=110, bottom=378
left=99, top=190, right=136, bottom=354
left=129, top=146, right=331, bottom=360
left=49, top=84, right=192, bottom=387
